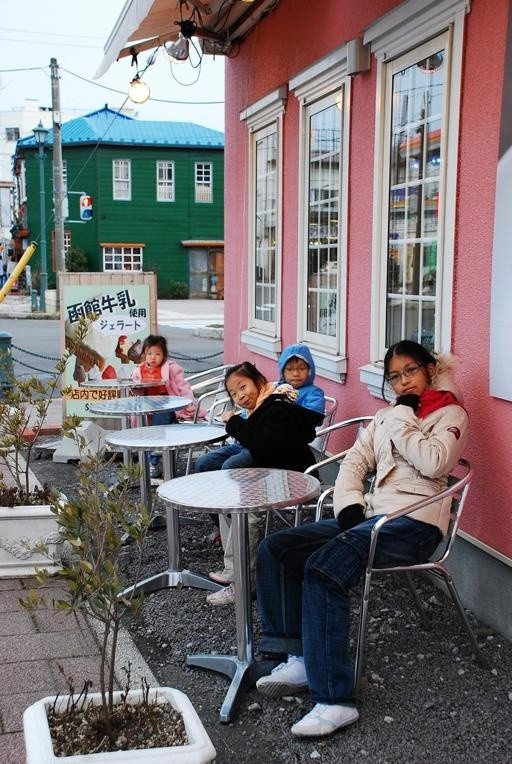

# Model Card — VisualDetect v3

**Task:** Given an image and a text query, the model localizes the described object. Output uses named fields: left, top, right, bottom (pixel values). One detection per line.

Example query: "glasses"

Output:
left=386, top=361, right=423, bottom=385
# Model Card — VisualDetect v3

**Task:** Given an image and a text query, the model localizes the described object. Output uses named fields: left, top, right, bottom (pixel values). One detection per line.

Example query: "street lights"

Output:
left=28, top=119, right=53, bottom=314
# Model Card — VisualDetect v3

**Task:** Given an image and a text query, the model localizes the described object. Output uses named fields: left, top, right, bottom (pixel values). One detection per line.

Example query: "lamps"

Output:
left=163, top=29, right=191, bottom=60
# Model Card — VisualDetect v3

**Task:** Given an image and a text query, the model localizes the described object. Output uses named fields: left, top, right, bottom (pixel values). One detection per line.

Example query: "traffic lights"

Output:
left=79, top=196, right=93, bottom=221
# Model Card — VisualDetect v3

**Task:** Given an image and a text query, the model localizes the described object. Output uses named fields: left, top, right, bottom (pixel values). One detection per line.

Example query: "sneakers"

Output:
left=256, top=659, right=309, bottom=696
left=211, top=528, right=222, bottom=545
left=209, top=571, right=234, bottom=584
left=291, top=703, right=359, bottom=736
left=206, top=582, right=236, bottom=606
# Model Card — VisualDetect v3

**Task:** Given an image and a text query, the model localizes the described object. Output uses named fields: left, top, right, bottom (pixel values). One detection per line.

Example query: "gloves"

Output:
left=338, top=505, right=365, bottom=531
left=394, top=394, right=420, bottom=412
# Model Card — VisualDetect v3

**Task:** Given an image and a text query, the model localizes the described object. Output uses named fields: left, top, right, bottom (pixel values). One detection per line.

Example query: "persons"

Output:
left=129, top=333, right=211, bottom=480
left=257, top=340, right=470, bottom=743
left=193, top=343, right=327, bottom=547
left=202, top=357, right=321, bottom=606
